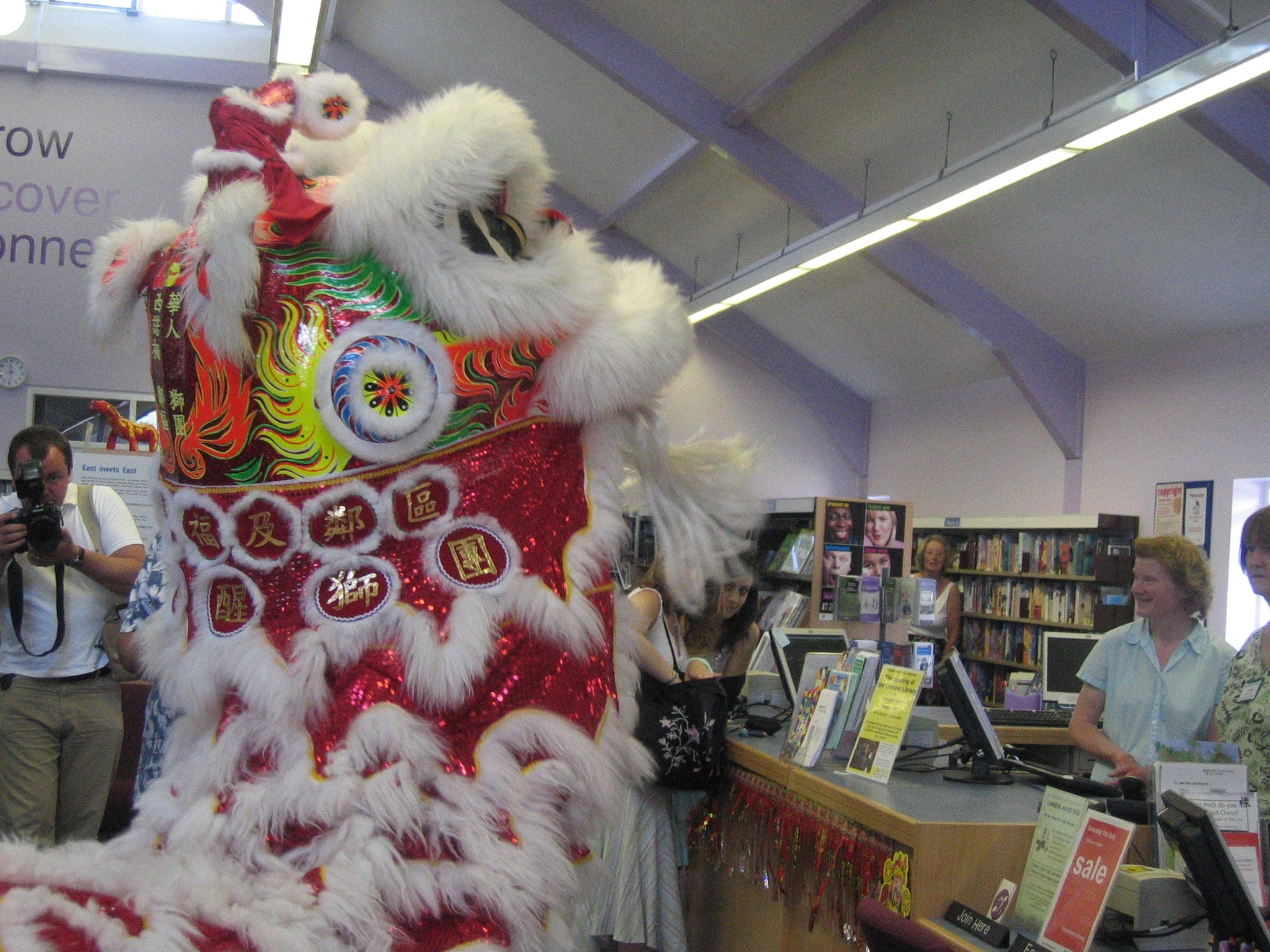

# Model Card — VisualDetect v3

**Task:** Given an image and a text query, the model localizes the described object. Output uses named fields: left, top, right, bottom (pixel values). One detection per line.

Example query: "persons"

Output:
left=626, top=539, right=725, bottom=698
left=1065, top=535, right=1238, bottom=789
left=0, top=424, right=147, bottom=851
left=909, top=535, right=961, bottom=667
left=821, top=545, right=852, bottom=588
left=863, top=508, right=904, bottom=549
left=118, top=515, right=187, bottom=818
left=863, top=547, right=891, bottom=578
left=1210, top=506, right=1270, bottom=841
left=824, top=506, right=854, bottom=545
left=678, top=554, right=760, bottom=678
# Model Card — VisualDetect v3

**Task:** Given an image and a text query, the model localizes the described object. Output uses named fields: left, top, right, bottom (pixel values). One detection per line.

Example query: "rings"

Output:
left=36, top=556, right=40, bottom=562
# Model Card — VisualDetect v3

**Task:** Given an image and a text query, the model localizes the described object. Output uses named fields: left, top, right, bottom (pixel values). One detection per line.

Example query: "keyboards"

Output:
left=998, top=756, right=1123, bottom=798
left=988, top=709, right=1104, bottom=727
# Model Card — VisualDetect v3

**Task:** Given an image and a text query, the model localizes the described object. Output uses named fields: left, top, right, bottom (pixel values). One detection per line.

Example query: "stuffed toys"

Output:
left=0, top=71, right=768, bottom=952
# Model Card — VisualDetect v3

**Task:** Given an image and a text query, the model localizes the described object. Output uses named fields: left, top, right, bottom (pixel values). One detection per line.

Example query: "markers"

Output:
left=747, top=732, right=769, bottom=737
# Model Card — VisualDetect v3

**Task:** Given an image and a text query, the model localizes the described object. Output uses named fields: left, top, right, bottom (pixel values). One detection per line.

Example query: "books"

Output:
left=738, top=519, right=1101, bottom=784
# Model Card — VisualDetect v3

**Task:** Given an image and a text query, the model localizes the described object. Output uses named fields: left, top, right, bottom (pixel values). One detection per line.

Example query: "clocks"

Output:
left=0, top=354, right=30, bottom=390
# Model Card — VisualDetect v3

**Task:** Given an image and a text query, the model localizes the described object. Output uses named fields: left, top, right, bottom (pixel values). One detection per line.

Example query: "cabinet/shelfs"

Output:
left=611, top=497, right=1139, bottom=708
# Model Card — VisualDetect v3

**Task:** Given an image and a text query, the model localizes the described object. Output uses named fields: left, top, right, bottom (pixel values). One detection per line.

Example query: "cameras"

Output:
left=9, top=460, right=63, bottom=555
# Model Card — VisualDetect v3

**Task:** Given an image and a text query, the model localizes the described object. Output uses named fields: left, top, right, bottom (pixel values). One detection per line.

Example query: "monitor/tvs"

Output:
left=1154, top=788, right=1270, bottom=952
left=771, top=628, right=849, bottom=708
left=933, top=647, right=1014, bottom=786
left=1042, top=632, right=1102, bottom=704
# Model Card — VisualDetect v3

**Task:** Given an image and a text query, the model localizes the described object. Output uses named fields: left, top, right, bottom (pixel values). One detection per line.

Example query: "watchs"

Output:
left=66, top=547, right=85, bottom=569
left=664, top=669, right=679, bottom=685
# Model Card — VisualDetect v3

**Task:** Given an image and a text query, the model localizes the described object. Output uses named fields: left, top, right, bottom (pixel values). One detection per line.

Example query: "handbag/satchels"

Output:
left=98, top=602, right=144, bottom=682
left=637, top=675, right=731, bottom=797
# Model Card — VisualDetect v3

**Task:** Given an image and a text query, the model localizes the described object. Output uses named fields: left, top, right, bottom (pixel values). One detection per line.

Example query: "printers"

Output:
left=1105, top=864, right=1210, bottom=950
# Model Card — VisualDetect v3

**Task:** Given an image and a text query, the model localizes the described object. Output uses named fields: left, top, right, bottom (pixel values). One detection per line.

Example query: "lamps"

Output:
left=683, top=0, right=1270, bottom=324
